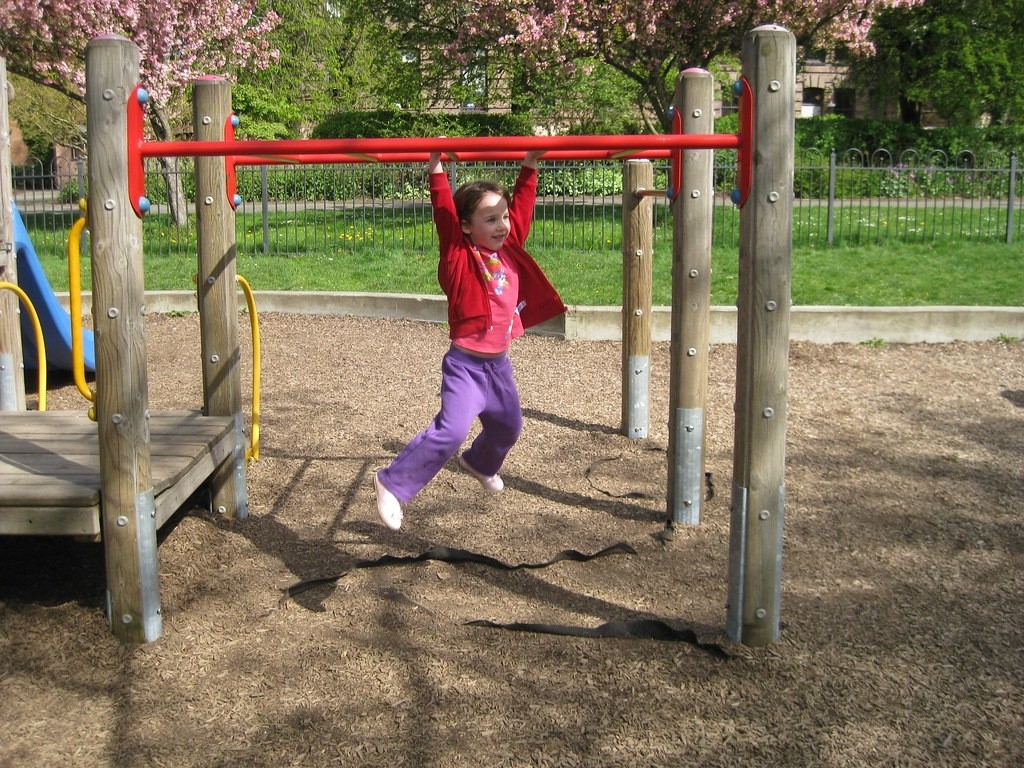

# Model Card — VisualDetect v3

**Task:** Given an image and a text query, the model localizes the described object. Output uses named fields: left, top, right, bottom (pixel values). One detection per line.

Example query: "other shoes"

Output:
left=373, top=473, right=404, bottom=530
left=459, top=454, right=504, bottom=491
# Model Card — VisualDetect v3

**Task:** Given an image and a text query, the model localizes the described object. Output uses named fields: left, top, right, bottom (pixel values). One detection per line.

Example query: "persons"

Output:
left=373, top=135, right=568, bottom=531
